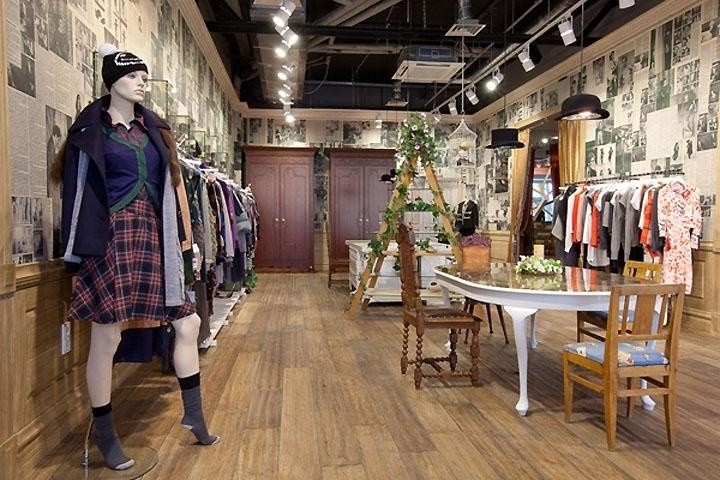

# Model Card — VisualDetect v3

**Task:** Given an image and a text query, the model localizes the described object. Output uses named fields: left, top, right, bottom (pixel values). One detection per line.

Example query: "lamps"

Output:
left=385, top=77, right=410, bottom=107
left=482, top=0, right=527, bottom=150
left=433, top=1, right=637, bottom=123
left=272, top=0, right=302, bottom=125
left=552, top=1, right=610, bottom=124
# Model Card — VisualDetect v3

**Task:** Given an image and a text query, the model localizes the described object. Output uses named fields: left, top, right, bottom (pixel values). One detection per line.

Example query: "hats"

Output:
left=483, top=128, right=525, bottom=149
left=98, top=42, right=150, bottom=92
left=553, top=93, right=610, bottom=120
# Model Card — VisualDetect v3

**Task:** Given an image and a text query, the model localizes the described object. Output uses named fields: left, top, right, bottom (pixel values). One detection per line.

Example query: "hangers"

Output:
left=558, top=167, right=688, bottom=191
left=175, top=144, right=252, bottom=197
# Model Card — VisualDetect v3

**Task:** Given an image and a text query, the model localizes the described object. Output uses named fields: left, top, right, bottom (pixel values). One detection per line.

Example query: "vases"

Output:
left=454, top=245, right=492, bottom=266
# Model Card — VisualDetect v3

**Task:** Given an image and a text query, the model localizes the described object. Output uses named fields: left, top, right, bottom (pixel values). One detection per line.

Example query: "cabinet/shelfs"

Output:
left=344, top=239, right=468, bottom=306
left=328, top=147, right=400, bottom=273
left=240, top=143, right=318, bottom=274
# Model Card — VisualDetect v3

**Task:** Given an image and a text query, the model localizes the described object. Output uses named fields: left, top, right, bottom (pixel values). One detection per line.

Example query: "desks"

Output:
left=431, top=263, right=671, bottom=417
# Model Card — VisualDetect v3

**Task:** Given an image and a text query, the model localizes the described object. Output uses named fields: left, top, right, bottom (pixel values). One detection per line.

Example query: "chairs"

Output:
left=562, top=283, right=686, bottom=453
left=575, top=260, right=664, bottom=345
left=325, top=222, right=350, bottom=289
left=394, top=222, right=484, bottom=391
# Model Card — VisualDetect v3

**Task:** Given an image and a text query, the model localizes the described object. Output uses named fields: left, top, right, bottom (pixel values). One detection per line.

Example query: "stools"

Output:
left=457, top=296, right=510, bottom=345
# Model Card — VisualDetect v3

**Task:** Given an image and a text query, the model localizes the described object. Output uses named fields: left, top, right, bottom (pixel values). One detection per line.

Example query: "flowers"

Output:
left=451, top=232, right=493, bottom=247
left=514, top=255, right=566, bottom=277
left=392, top=108, right=441, bottom=176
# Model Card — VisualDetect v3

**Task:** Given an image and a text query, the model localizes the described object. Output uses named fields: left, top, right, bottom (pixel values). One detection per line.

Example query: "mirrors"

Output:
left=514, top=116, right=567, bottom=265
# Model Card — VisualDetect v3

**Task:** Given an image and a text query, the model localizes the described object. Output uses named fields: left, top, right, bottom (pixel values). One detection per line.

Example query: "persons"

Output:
left=55, top=42, right=220, bottom=471
left=570, top=50, right=720, bottom=177
left=455, top=200, right=479, bottom=236
